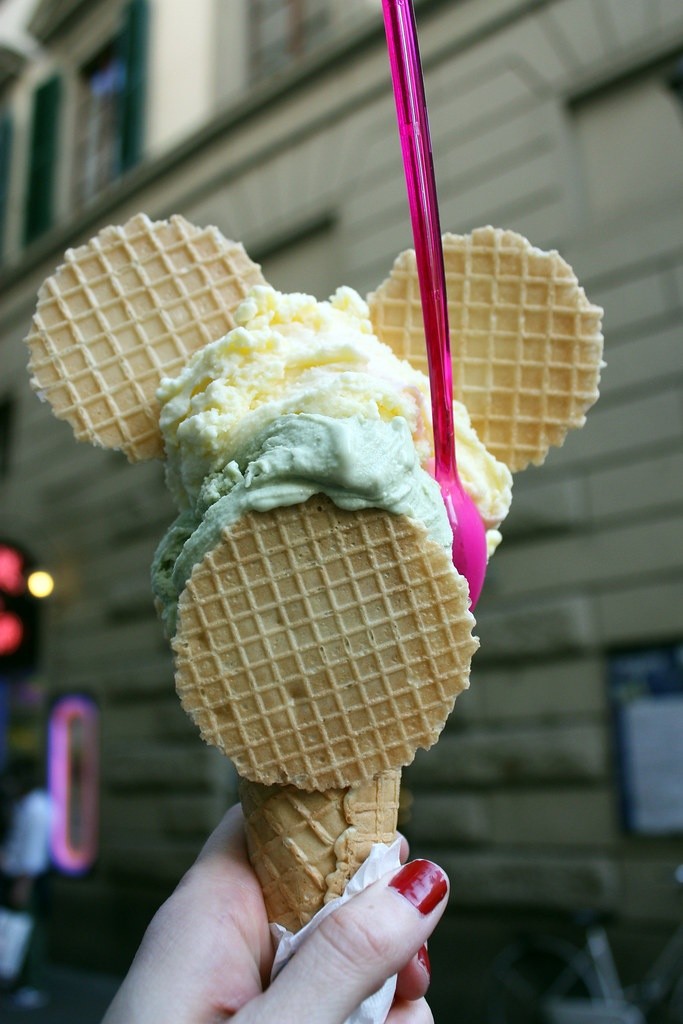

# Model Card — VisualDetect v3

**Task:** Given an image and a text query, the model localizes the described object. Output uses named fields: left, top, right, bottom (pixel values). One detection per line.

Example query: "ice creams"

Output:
left=24, top=212, right=607, bottom=942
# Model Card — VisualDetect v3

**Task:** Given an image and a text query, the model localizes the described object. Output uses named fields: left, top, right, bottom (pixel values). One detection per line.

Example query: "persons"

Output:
left=101, top=801, right=452, bottom=1023
